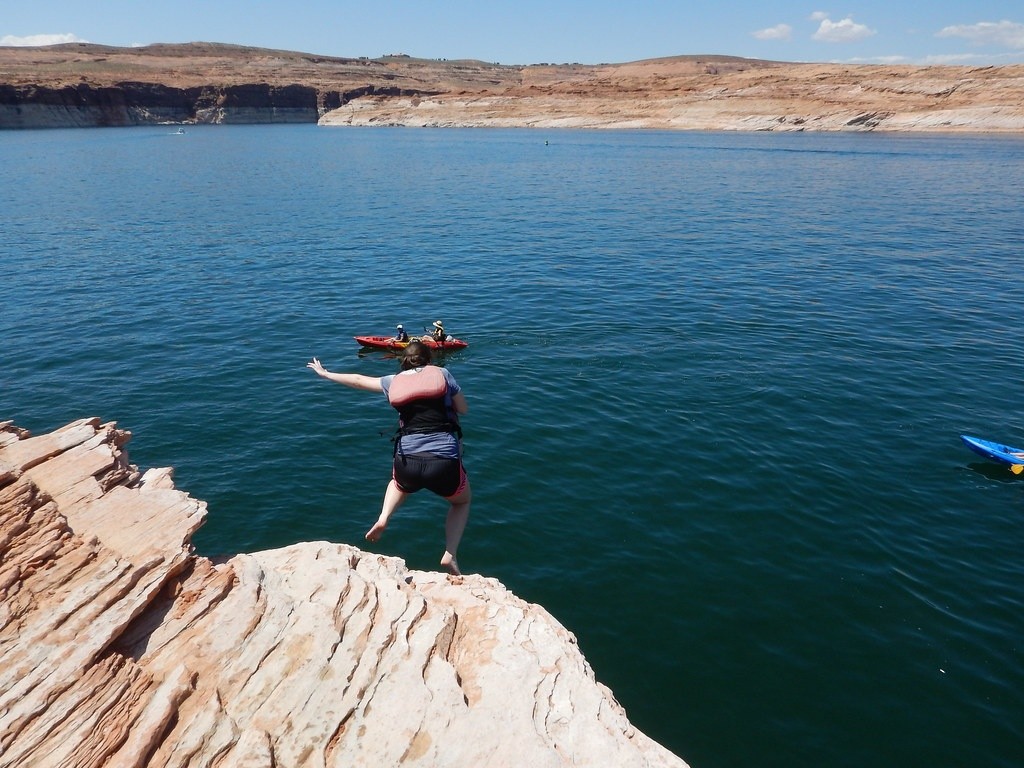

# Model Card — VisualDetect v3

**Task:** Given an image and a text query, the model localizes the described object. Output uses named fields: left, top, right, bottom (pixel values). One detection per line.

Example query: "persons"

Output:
left=389, top=325, right=410, bottom=342
left=423, top=320, right=444, bottom=342
left=307, top=339, right=471, bottom=575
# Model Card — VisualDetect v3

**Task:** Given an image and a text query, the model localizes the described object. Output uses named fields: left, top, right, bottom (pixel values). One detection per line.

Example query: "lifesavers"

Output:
left=387, top=365, right=448, bottom=406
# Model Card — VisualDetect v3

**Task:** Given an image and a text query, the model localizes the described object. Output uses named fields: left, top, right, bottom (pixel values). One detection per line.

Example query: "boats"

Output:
left=959, top=433, right=1024, bottom=475
left=354, top=334, right=469, bottom=351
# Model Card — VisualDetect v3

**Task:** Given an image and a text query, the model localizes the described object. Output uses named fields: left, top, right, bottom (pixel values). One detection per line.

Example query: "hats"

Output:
left=432, top=320, right=444, bottom=330
left=396, top=325, right=402, bottom=329
